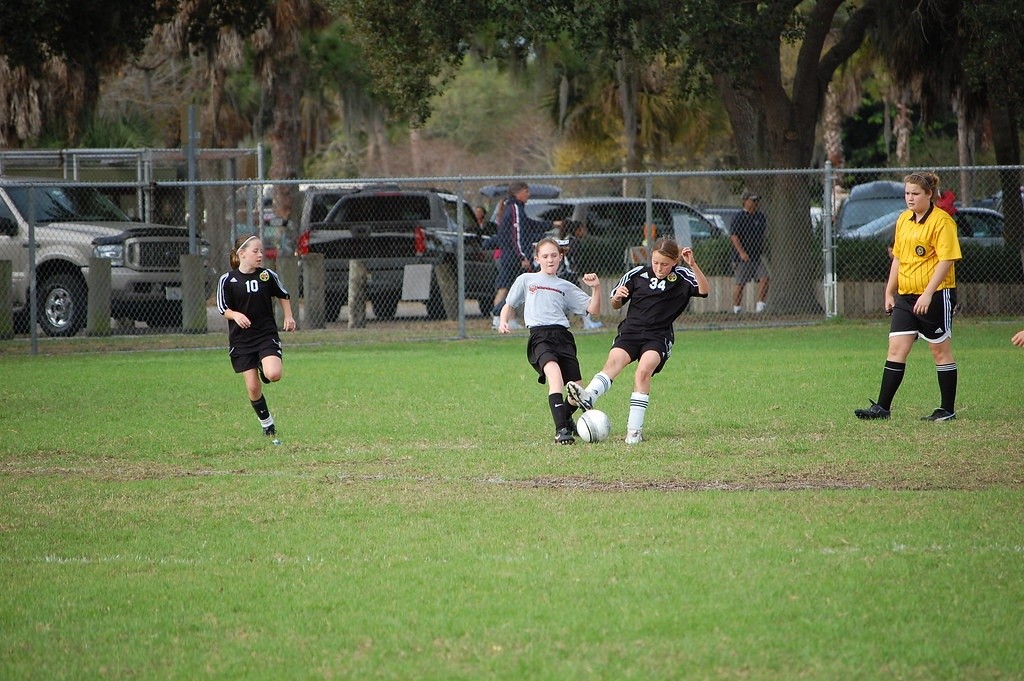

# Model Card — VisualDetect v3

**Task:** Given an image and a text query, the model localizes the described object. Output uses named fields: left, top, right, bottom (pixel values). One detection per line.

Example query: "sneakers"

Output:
left=566, top=381, right=594, bottom=412
left=854, top=398, right=891, bottom=420
left=258, top=362, right=271, bottom=386
left=625, top=431, right=644, bottom=444
left=921, top=407, right=957, bottom=423
left=554, top=427, right=575, bottom=446
left=262, top=425, right=277, bottom=439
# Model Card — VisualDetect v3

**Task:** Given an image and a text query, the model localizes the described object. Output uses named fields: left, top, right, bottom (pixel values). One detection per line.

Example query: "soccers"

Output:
left=576, top=409, right=611, bottom=444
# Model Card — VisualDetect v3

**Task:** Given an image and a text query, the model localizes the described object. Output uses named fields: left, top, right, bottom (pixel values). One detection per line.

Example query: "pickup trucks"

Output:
left=294, top=187, right=497, bottom=319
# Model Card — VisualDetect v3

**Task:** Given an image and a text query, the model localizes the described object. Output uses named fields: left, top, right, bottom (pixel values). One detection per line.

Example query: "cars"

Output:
left=184, top=184, right=400, bottom=228
left=688, top=208, right=741, bottom=240
left=985, top=187, right=1024, bottom=238
left=834, top=181, right=907, bottom=236
left=1, top=174, right=217, bottom=337
left=837, top=207, right=1005, bottom=282
left=482, top=196, right=743, bottom=275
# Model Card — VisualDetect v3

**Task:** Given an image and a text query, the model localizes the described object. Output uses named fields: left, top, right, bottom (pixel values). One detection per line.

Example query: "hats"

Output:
left=741, top=191, right=761, bottom=200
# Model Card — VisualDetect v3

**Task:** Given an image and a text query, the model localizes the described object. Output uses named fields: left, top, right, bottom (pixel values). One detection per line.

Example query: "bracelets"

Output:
left=613, top=296, right=621, bottom=302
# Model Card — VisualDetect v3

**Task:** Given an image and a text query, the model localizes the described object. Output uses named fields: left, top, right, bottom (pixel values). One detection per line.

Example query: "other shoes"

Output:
left=584, top=319, right=604, bottom=331
left=491, top=319, right=526, bottom=330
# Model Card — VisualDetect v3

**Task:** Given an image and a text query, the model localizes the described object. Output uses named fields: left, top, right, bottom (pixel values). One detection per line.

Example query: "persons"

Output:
left=463, top=179, right=605, bottom=331
left=727, top=190, right=773, bottom=315
left=853, top=170, right=963, bottom=422
left=886, top=241, right=963, bottom=318
left=214, top=233, right=298, bottom=447
left=566, top=239, right=710, bottom=445
left=498, top=238, right=601, bottom=445
left=1010, top=329, right=1024, bottom=350
